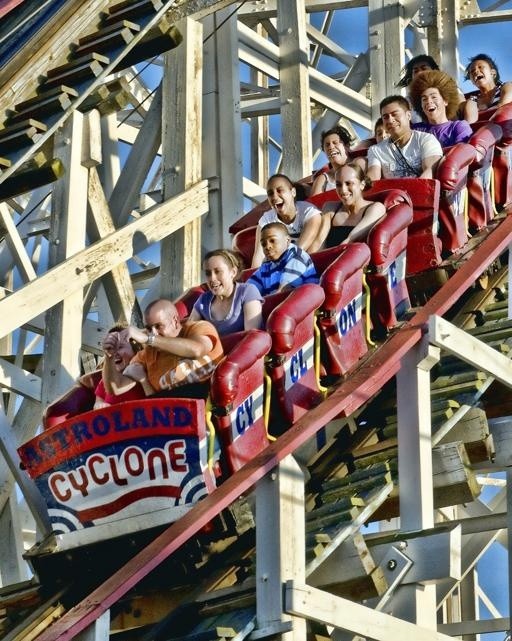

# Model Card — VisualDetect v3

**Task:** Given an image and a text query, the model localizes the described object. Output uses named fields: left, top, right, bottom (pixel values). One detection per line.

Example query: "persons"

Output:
left=189, top=247, right=265, bottom=337
left=251, top=162, right=386, bottom=268
left=93, top=298, right=225, bottom=403
left=310, top=52, right=511, bottom=195
left=244, top=221, right=319, bottom=297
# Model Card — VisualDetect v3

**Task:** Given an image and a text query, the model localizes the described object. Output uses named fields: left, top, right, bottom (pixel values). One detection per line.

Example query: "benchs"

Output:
left=42, top=102, right=512, bottom=476
